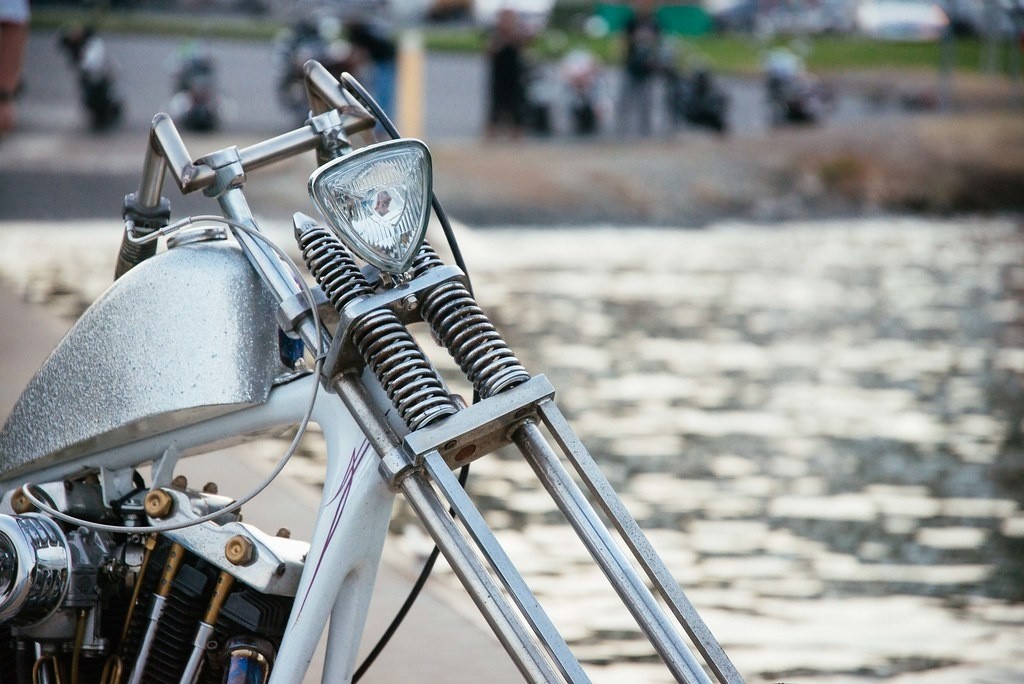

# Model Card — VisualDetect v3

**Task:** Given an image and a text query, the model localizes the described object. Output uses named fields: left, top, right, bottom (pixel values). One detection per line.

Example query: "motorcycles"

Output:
left=0, top=57, right=746, bottom=684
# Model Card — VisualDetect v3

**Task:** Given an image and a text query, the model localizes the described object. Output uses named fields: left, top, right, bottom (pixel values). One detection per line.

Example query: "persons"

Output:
left=0, top=0, right=834, bottom=140
